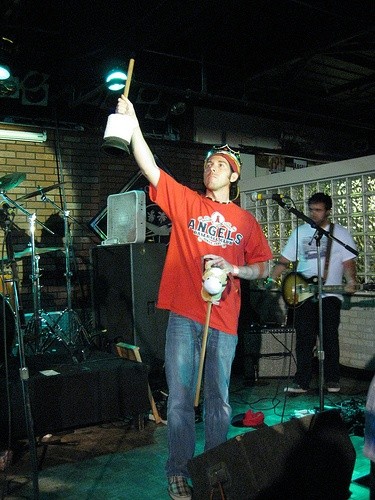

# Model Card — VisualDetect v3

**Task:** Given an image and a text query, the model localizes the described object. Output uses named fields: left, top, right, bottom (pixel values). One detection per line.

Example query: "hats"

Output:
left=204, top=144, right=242, bottom=180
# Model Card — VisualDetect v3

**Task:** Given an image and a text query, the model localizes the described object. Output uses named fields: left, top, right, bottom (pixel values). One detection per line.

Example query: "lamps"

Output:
left=0, top=129, right=47, bottom=143
left=0, top=64, right=50, bottom=107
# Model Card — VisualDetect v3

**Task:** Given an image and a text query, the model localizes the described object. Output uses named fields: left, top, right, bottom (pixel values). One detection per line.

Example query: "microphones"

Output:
left=250, top=192, right=287, bottom=201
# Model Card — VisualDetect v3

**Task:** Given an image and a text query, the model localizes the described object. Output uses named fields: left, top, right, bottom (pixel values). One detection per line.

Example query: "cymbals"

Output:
left=0, top=172, right=25, bottom=190
left=15, top=180, right=69, bottom=202
left=0, top=246, right=62, bottom=262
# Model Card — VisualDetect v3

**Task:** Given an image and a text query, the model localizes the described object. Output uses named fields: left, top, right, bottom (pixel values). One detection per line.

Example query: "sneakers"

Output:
left=167, top=475, right=191, bottom=500
left=284, top=383, right=308, bottom=393
left=327, top=382, right=340, bottom=392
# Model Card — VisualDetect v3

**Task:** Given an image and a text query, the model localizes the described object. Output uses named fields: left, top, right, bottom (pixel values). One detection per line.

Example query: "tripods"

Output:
left=0, top=131, right=100, bottom=367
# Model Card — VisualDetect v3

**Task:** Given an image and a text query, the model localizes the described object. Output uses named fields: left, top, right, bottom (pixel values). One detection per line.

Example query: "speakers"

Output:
left=241, top=322, right=299, bottom=378
left=91, top=242, right=167, bottom=381
left=187, top=408, right=356, bottom=500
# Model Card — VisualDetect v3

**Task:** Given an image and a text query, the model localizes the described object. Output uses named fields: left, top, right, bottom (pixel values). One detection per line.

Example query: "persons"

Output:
left=116, top=93, right=272, bottom=500
left=263, top=191, right=358, bottom=395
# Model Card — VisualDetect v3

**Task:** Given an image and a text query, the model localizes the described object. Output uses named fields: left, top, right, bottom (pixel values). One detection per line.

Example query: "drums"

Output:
left=0, top=271, right=20, bottom=312
left=0, top=294, right=18, bottom=363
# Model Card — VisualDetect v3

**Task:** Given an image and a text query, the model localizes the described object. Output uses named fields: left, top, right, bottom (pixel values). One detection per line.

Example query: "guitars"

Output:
left=281, top=271, right=375, bottom=309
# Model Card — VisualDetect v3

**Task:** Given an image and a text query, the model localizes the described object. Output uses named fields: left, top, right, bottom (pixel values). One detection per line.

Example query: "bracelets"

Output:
left=231, top=264, right=239, bottom=277
